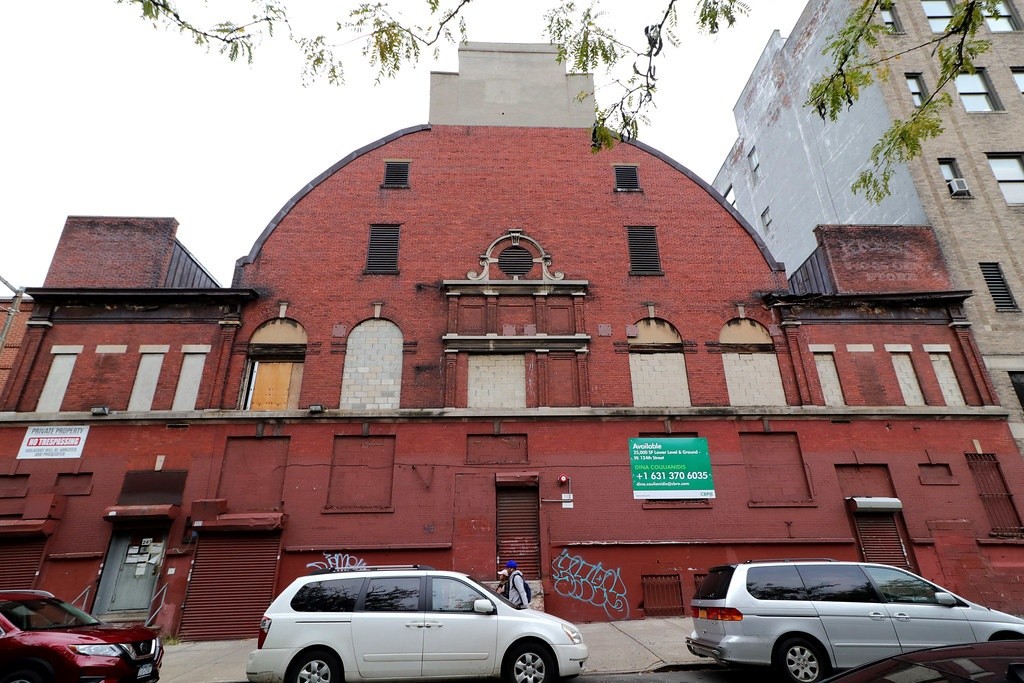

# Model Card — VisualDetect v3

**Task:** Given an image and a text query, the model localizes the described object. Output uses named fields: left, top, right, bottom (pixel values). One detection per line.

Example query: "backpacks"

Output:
left=511, top=574, right=531, bottom=603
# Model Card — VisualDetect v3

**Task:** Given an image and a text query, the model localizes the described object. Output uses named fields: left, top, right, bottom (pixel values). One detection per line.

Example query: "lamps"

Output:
left=89, top=406, right=109, bottom=415
left=309, top=405, right=324, bottom=413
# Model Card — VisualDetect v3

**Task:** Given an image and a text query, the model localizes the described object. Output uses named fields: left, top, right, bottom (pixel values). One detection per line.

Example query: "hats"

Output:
left=498, top=570, right=508, bottom=576
left=506, top=561, right=517, bottom=568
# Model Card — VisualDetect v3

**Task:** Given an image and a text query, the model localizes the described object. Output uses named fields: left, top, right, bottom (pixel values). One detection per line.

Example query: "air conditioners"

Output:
left=948, top=178, right=968, bottom=197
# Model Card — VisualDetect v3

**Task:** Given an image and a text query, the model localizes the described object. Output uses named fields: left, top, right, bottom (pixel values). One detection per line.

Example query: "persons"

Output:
left=496, top=569, right=510, bottom=600
left=506, top=561, right=530, bottom=609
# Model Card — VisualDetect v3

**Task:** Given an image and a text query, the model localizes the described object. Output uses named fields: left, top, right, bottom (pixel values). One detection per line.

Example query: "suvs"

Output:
left=0, top=589, right=164, bottom=683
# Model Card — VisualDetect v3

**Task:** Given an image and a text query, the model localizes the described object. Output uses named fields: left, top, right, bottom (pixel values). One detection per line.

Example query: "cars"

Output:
left=246, top=563, right=590, bottom=683
left=814, top=639, right=1024, bottom=683
left=684, top=557, right=1024, bottom=683
